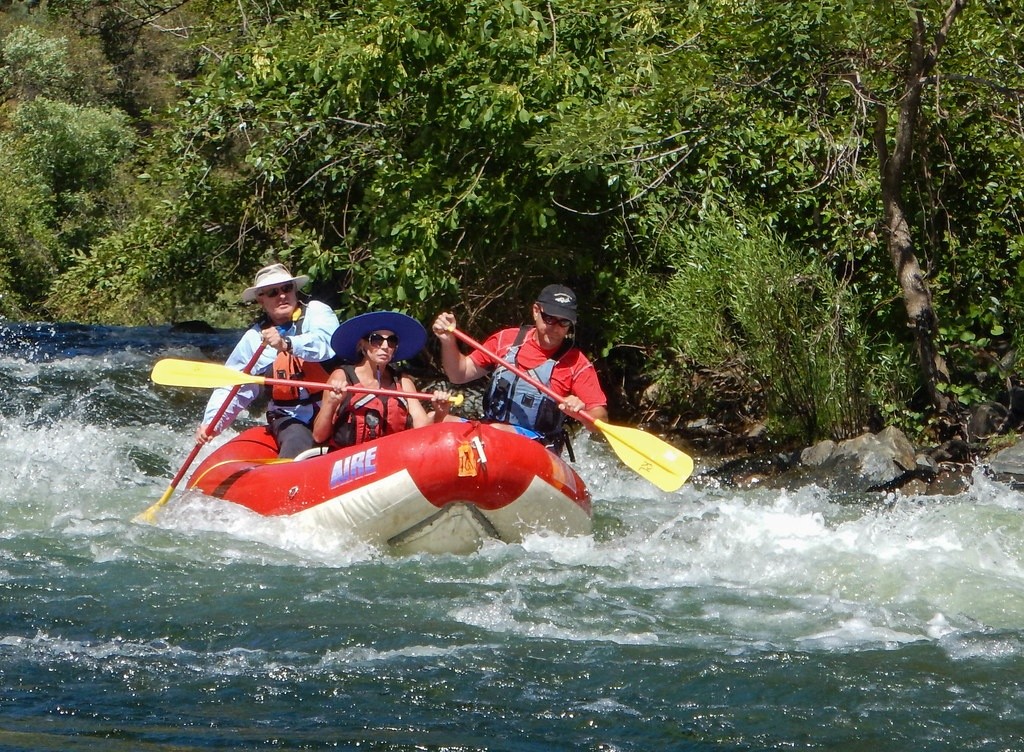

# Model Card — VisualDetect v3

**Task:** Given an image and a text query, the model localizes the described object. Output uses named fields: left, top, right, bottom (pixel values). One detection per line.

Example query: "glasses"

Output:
left=259, top=283, right=294, bottom=297
left=539, top=311, right=572, bottom=327
left=361, top=332, right=399, bottom=348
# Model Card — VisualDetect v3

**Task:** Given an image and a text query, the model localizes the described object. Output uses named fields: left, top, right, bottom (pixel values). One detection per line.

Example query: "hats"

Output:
left=535, top=284, right=578, bottom=322
left=330, top=311, right=428, bottom=362
left=242, top=263, right=309, bottom=301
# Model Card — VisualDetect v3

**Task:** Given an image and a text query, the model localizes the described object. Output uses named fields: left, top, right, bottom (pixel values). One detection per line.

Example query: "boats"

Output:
left=185, top=425, right=592, bottom=555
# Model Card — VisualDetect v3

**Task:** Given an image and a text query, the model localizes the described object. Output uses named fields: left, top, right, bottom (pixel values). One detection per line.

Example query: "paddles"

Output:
left=150, top=358, right=466, bottom=406
left=448, top=325, right=694, bottom=494
left=130, top=340, right=271, bottom=523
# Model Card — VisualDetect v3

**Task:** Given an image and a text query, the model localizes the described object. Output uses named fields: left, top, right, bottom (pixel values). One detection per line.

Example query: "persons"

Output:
left=196, top=266, right=452, bottom=466
left=434, top=283, right=607, bottom=451
